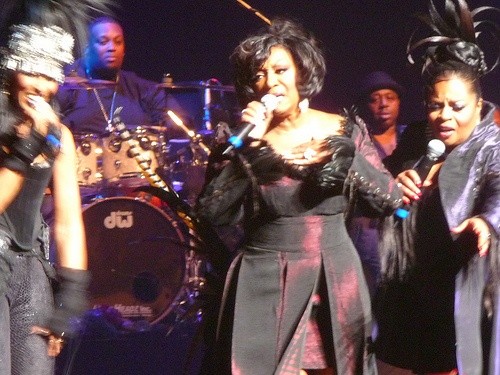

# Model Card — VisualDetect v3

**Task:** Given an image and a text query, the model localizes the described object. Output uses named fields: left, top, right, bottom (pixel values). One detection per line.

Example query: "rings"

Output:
left=488, top=233, right=491, bottom=240
left=48, top=339, right=64, bottom=343
left=303, top=147, right=317, bottom=160
left=397, top=182, right=402, bottom=188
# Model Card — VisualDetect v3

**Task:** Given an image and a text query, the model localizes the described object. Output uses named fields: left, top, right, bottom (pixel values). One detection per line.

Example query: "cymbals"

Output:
left=154, top=78, right=202, bottom=92
left=60, top=77, right=117, bottom=88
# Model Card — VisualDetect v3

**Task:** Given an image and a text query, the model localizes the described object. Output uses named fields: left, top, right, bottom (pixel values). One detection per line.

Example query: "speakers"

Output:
left=59, top=307, right=207, bottom=375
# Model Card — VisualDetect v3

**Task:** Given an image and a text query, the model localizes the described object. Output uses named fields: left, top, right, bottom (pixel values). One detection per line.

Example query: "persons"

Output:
left=344, top=70, right=410, bottom=288
left=372, top=0, right=500, bottom=375
left=0, top=0, right=118, bottom=375
left=196, top=18, right=403, bottom=375
left=57, top=16, right=190, bottom=143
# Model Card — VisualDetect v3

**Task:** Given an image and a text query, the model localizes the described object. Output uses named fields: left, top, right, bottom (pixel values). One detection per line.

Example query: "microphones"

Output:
left=28, top=94, right=61, bottom=153
left=395, top=139, right=445, bottom=219
left=221, top=93, right=278, bottom=156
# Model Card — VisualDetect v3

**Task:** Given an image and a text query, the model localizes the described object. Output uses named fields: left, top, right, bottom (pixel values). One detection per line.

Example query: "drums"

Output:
left=99, top=120, right=170, bottom=192
left=42, top=126, right=103, bottom=196
left=44, top=183, right=193, bottom=326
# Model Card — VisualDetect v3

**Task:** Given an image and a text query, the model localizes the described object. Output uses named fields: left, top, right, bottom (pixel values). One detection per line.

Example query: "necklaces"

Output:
left=86, top=70, right=120, bottom=132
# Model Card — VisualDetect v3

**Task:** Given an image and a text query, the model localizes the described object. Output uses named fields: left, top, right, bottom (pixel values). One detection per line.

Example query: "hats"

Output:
left=361, top=70, right=404, bottom=98
